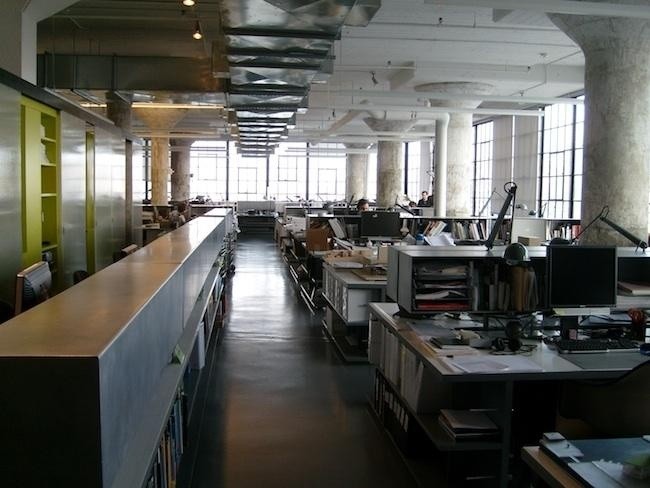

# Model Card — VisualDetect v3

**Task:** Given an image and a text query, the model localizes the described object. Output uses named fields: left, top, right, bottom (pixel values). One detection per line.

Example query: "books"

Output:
left=367, top=321, right=450, bottom=433
left=421, top=220, right=506, bottom=242
left=438, top=408, right=500, bottom=440
left=414, top=263, right=538, bottom=312
left=549, top=224, right=582, bottom=242
left=322, top=257, right=388, bottom=323
left=421, top=337, right=510, bottom=373
left=345, top=335, right=361, bottom=346
left=615, top=280, right=650, bottom=310
left=145, top=383, right=187, bottom=488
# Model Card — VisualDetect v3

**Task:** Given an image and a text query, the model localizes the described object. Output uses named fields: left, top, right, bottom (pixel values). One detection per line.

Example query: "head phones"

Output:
left=491, top=334, right=524, bottom=353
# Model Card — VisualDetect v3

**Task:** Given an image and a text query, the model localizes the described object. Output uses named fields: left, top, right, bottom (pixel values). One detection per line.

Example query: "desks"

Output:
left=236, top=211, right=275, bottom=235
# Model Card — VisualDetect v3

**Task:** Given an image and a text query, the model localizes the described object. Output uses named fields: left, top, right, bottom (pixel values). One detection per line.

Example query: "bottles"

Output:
left=417, top=234, right=424, bottom=244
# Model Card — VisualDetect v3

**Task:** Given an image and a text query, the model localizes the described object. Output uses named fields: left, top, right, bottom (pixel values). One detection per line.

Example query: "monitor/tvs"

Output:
left=361, top=209, right=403, bottom=243
left=14, top=260, right=54, bottom=315
left=328, top=207, right=348, bottom=214
left=542, top=241, right=619, bottom=342
left=115, top=242, right=139, bottom=255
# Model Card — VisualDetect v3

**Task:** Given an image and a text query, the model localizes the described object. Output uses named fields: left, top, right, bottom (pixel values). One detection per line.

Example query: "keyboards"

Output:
left=555, top=338, right=639, bottom=355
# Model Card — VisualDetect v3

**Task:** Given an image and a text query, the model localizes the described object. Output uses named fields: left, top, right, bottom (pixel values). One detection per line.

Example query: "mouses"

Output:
left=638, top=342, right=649, bottom=354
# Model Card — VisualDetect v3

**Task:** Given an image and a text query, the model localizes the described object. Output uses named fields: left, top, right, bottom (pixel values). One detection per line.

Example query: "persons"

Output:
left=357, top=199, right=370, bottom=213
left=418, top=191, right=433, bottom=207
left=409, top=201, right=417, bottom=208
left=164, top=204, right=186, bottom=226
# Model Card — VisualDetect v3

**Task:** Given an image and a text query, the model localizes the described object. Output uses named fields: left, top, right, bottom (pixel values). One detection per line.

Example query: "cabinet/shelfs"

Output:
left=117, top=230, right=236, bottom=487
left=21, top=103, right=59, bottom=290
